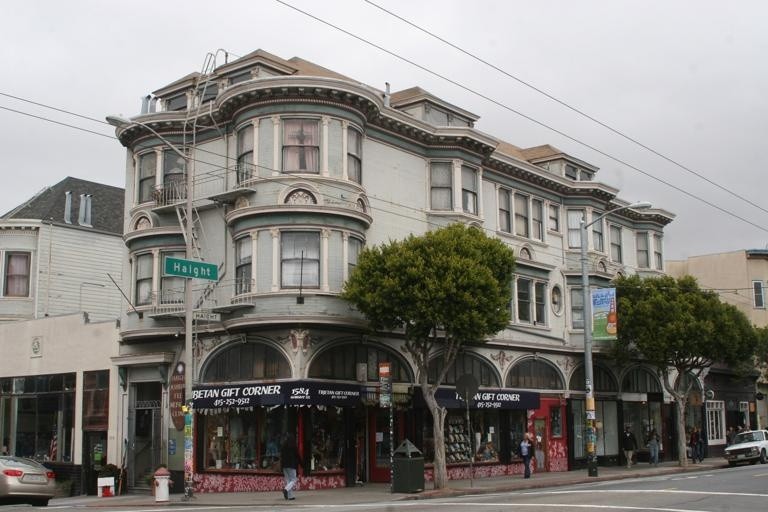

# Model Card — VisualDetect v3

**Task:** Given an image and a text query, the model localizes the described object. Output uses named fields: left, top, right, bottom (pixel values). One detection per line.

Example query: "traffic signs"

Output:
left=163, top=257, right=219, bottom=281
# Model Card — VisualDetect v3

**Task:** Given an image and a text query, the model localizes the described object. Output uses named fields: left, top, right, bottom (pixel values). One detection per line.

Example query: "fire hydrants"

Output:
left=152, top=467, right=174, bottom=502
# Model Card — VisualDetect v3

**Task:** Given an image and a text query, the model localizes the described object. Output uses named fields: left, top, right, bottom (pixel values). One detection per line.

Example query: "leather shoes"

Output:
left=283, top=489, right=287, bottom=499
left=288, top=498, right=294, bottom=500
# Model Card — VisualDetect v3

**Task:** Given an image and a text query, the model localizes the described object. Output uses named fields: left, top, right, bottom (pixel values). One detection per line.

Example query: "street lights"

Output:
left=106, top=115, right=196, bottom=501
left=580, top=200, right=651, bottom=477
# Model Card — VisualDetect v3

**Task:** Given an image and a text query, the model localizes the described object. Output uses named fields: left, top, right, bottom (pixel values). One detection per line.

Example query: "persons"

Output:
left=645, top=428, right=662, bottom=468
left=280, top=431, right=306, bottom=501
left=621, top=426, right=639, bottom=469
left=0, top=446, right=9, bottom=456
left=517, top=432, right=535, bottom=479
left=689, top=424, right=749, bottom=465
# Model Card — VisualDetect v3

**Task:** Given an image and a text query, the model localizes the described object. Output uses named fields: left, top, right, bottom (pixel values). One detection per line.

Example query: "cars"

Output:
left=724, top=429, right=768, bottom=466
left=0, top=454, right=55, bottom=507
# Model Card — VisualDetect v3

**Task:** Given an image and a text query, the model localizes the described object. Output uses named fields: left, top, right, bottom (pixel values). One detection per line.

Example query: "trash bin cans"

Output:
left=393, top=439, right=424, bottom=493
